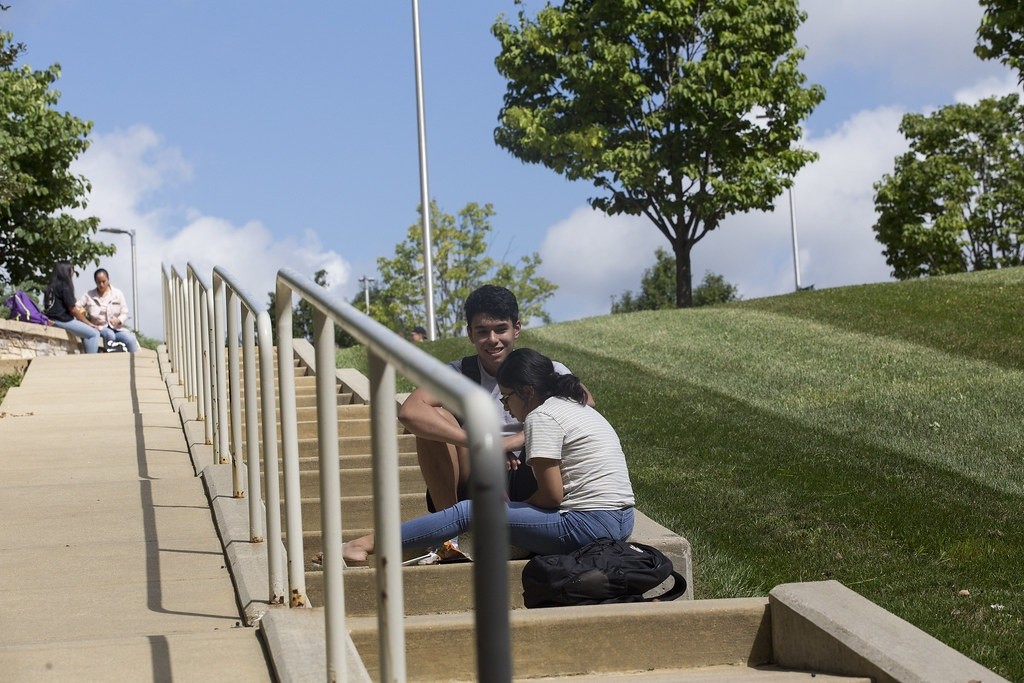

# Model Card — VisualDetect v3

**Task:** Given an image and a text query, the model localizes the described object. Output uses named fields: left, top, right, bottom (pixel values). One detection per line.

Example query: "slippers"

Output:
left=311, top=541, right=369, bottom=568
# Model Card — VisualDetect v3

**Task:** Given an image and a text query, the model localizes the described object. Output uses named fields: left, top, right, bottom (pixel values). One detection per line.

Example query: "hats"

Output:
left=412, top=327, right=428, bottom=340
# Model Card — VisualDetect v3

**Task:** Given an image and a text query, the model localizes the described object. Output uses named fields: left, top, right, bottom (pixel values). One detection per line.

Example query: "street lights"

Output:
left=755, top=113, right=803, bottom=291
left=100, top=228, right=139, bottom=335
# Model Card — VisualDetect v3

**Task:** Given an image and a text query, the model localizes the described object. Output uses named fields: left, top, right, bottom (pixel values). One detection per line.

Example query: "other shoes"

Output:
left=418, top=538, right=460, bottom=565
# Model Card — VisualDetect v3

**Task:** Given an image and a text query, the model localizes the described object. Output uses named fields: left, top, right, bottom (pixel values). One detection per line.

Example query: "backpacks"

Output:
left=522, top=539, right=687, bottom=610
left=7, top=291, right=49, bottom=329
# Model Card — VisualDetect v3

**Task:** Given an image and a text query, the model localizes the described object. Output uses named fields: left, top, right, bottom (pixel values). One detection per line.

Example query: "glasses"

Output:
left=500, top=390, right=516, bottom=410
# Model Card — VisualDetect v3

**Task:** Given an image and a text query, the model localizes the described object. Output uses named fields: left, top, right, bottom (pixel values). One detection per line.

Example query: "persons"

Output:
left=310, top=348, right=636, bottom=570
left=44, top=260, right=100, bottom=353
left=398, top=285, right=595, bottom=565
left=75, top=269, right=140, bottom=353
left=410, top=327, right=427, bottom=343
